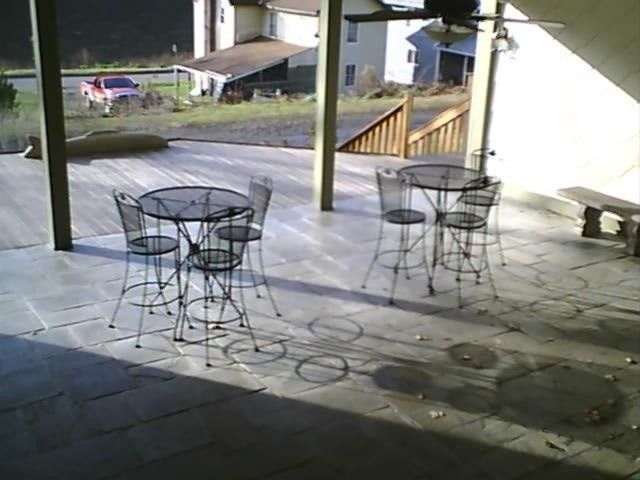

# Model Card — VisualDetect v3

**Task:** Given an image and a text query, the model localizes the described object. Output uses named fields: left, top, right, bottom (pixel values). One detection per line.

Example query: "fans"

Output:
left=344, top=0, right=567, bottom=39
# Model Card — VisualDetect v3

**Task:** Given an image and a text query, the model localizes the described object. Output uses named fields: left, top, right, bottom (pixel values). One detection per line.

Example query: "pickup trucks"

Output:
left=80, top=73, right=146, bottom=114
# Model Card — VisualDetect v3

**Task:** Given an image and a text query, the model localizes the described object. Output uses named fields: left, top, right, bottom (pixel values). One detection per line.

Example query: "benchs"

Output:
left=561, top=183, right=638, bottom=251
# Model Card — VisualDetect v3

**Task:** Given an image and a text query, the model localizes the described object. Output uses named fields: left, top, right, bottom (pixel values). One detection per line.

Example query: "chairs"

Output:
left=111, top=175, right=274, bottom=368
left=362, top=163, right=504, bottom=304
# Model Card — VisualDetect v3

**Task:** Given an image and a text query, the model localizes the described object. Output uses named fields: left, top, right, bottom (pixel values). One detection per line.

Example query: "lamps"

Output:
left=488, top=21, right=512, bottom=53
left=423, top=11, right=480, bottom=47
